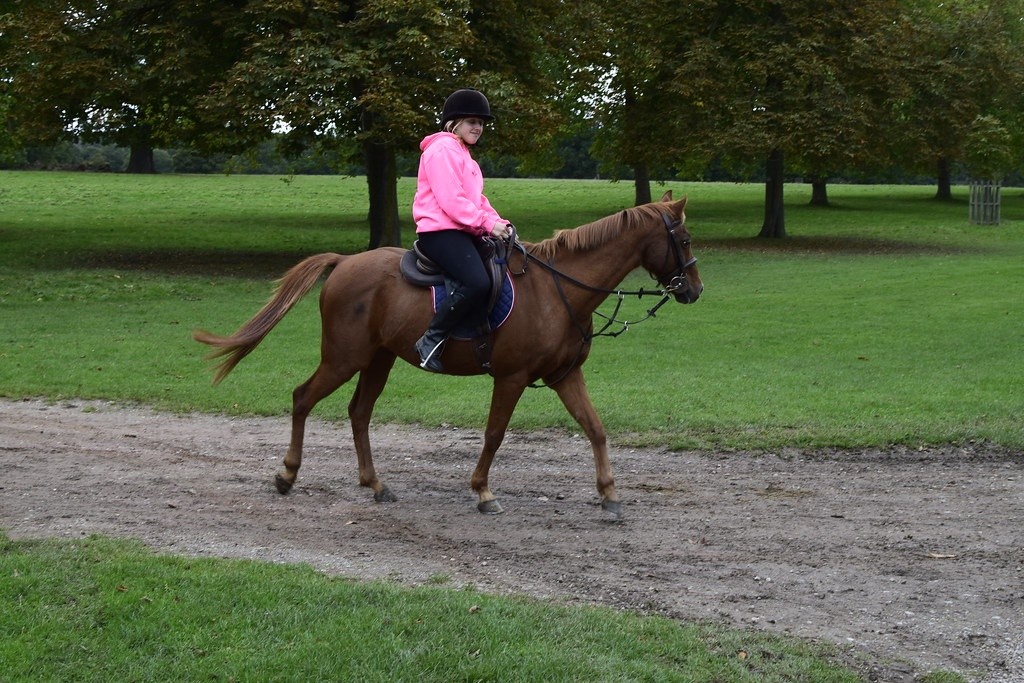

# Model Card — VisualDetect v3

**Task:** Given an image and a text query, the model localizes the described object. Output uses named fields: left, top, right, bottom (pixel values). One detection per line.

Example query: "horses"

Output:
left=193, top=190, right=704, bottom=514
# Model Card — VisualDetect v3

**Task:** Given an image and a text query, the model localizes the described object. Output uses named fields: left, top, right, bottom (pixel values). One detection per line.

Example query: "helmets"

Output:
left=440, top=88, right=494, bottom=127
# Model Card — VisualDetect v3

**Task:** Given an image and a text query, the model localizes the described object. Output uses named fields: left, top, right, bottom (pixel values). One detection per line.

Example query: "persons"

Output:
left=412, top=88, right=514, bottom=371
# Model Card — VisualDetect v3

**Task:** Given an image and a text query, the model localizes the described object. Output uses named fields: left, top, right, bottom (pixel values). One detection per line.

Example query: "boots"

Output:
left=414, top=287, right=470, bottom=371
